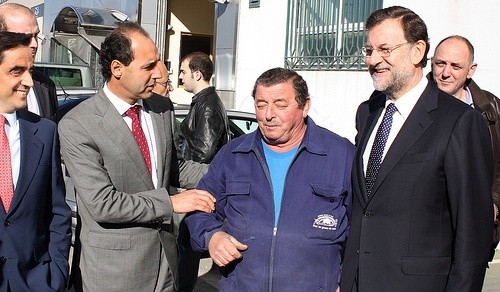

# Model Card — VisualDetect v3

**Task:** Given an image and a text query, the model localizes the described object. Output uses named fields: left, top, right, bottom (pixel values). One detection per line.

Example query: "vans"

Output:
left=34, top=63, right=91, bottom=94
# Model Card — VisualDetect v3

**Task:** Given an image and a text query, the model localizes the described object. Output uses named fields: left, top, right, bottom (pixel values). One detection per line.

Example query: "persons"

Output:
left=178, top=52, right=230, bottom=292
left=186, top=67, right=357, bottom=292
left=58, top=22, right=217, bottom=292
left=0, top=31, right=73, bottom=292
left=339, top=6, right=495, bottom=292
left=152, top=61, right=173, bottom=98
left=425, top=36, right=500, bottom=292
left=0, top=3, right=59, bottom=122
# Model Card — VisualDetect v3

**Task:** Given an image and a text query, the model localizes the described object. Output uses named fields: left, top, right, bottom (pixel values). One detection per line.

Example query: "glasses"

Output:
left=361, top=41, right=410, bottom=58
left=156, top=79, right=170, bottom=86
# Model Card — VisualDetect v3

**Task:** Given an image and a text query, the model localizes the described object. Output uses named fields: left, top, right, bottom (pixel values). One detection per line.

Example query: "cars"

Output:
left=55, top=97, right=257, bottom=141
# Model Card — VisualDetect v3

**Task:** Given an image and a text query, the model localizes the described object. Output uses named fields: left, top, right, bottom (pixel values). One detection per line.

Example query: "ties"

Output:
left=125, top=106, right=152, bottom=179
left=0, top=114, right=15, bottom=213
left=365, top=103, right=397, bottom=198
